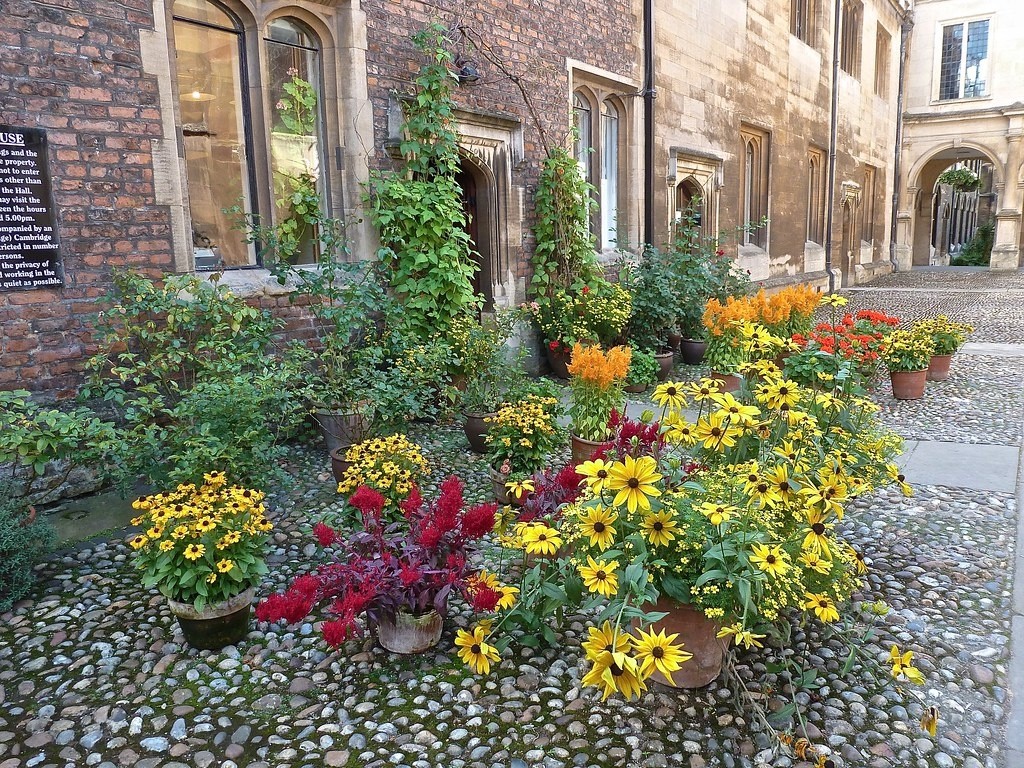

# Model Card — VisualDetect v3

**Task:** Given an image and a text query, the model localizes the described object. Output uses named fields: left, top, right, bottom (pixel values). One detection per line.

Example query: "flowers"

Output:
left=452, top=282, right=938, bottom=767
left=336, top=432, right=431, bottom=492
left=130, top=471, right=272, bottom=615
left=255, top=474, right=500, bottom=655
left=914, top=314, right=973, bottom=355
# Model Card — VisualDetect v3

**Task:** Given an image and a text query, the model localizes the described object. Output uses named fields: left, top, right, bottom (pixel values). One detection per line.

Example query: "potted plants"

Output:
left=955, top=178, right=983, bottom=193
left=0, top=389, right=111, bottom=528
left=643, top=334, right=674, bottom=382
left=623, top=337, right=661, bottom=392
left=438, top=321, right=466, bottom=406
left=310, top=349, right=371, bottom=450
left=530, top=147, right=571, bottom=380
left=939, top=169, right=974, bottom=187
left=680, top=318, right=707, bottom=365
left=657, top=303, right=685, bottom=349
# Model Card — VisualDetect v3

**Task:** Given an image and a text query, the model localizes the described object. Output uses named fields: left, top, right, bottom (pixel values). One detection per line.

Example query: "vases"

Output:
left=570, top=428, right=617, bottom=464
left=377, top=607, right=442, bottom=655
left=490, top=463, right=541, bottom=505
left=627, top=594, right=735, bottom=687
left=926, top=355, right=951, bottom=382
left=888, top=368, right=928, bottom=399
left=329, top=445, right=353, bottom=484
left=168, top=585, right=255, bottom=652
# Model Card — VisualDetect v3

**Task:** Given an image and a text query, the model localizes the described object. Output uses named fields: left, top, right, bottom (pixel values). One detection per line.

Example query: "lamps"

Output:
left=461, top=67, right=481, bottom=82
